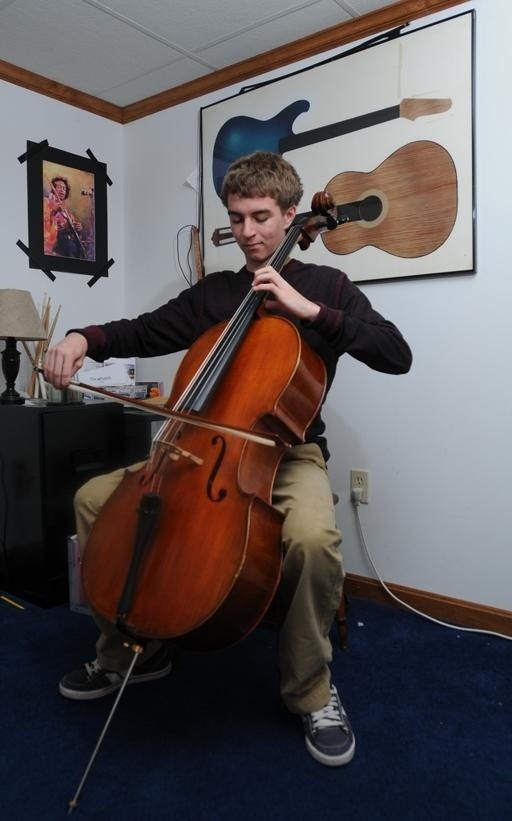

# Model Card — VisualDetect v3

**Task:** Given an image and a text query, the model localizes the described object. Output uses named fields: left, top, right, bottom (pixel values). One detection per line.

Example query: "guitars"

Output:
left=211, top=140, right=457, bottom=258
left=213, top=98, right=452, bottom=198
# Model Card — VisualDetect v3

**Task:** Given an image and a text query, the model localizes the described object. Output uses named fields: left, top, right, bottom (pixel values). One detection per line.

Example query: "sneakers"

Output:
left=300, top=682, right=357, bottom=768
left=57, top=652, right=172, bottom=702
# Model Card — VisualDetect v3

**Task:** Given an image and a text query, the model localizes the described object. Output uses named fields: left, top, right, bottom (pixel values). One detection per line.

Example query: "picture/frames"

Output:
left=26, top=141, right=108, bottom=277
left=199, top=8, right=476, bottom=281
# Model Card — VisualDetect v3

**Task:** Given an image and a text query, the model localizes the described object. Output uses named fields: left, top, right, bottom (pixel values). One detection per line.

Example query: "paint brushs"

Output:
left=22, top=292, right=62, bottom=399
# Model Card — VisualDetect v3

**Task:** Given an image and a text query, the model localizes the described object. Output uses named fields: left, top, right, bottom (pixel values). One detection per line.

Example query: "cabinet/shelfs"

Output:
left=2, top=403, right=166, bottom=610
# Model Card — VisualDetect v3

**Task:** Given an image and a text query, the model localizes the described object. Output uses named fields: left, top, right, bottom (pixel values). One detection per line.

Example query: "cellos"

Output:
left=81, top=193, right=337, bottom=654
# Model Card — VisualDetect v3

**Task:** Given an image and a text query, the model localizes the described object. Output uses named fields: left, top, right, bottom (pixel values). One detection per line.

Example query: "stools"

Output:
left=334, top=490, right=347, bottom=648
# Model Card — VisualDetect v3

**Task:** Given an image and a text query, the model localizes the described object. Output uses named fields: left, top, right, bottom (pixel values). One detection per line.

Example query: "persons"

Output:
left=42, top=177, right=86, bottom=260
left=36, top=147, right=414, bottom=770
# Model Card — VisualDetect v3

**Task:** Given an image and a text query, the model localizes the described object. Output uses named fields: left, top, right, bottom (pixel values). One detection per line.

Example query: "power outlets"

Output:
left=350, top=470, right=369, bottom=504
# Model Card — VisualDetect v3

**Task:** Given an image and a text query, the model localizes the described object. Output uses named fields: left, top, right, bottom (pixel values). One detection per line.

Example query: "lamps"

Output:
left=0, top=289, right=47, bottom=404
